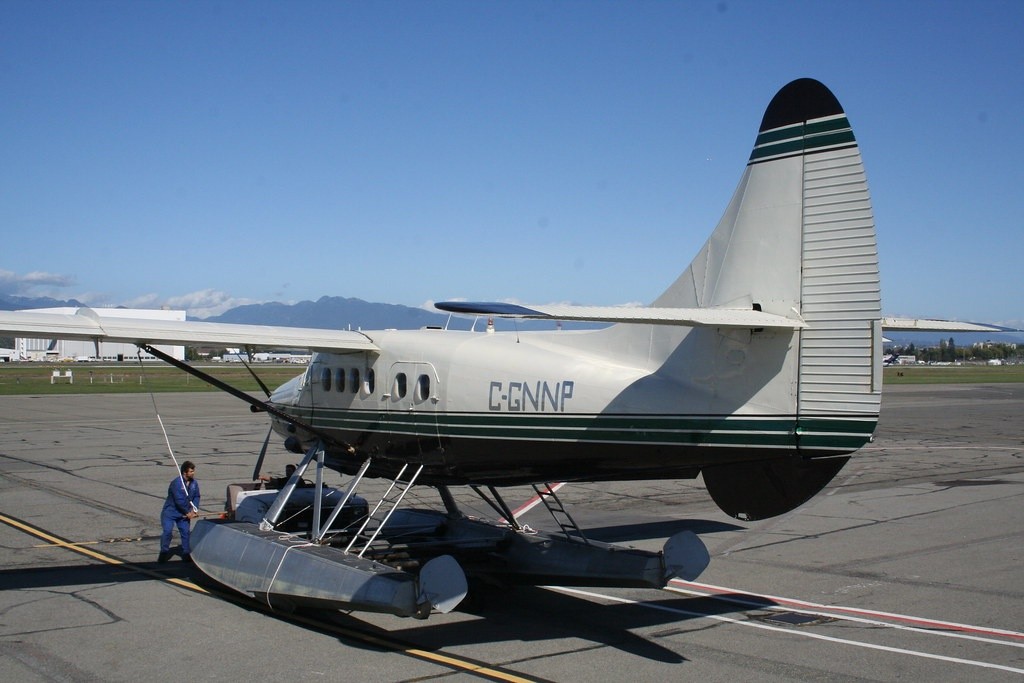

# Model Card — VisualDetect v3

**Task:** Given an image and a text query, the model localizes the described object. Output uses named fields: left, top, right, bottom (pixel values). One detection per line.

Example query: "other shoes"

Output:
left=159, top=559, right=168, bottom=567
left=182, top=554, right=190, bottom=561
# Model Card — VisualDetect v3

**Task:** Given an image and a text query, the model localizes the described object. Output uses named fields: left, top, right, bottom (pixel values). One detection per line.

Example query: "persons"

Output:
left=159, top=461, right=200, bottom=563
left=260, top=465, right=305, bottom=488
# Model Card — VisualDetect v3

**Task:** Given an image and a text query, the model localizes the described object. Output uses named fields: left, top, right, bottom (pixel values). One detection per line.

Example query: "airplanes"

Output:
left=0, top=75, right=1024, bottom=622
